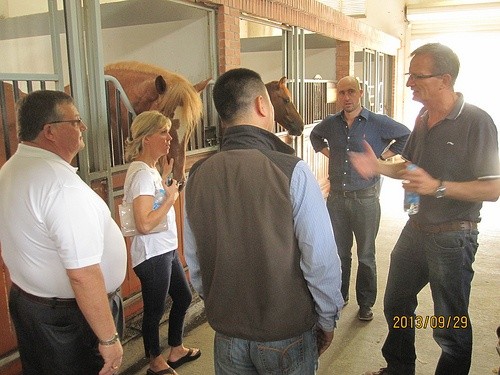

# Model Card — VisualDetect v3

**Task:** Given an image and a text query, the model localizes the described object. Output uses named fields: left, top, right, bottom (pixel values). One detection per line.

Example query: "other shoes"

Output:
left=361, top=368, right=386, bottom=375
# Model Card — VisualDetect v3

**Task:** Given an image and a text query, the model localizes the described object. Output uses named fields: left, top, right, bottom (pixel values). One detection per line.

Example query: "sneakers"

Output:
left=359, top=307, right=373, bottom=320
left=344, top=301, right=348, bottom=306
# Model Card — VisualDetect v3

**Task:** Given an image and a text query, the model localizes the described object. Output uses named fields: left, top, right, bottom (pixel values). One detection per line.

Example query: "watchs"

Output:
left=99, top=332, right=119, bottom=345
left=433, top=178, right=446, bottom=199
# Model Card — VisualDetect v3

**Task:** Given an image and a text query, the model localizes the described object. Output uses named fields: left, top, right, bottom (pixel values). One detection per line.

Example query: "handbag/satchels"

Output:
left=119, top=169, right=168, bottom=237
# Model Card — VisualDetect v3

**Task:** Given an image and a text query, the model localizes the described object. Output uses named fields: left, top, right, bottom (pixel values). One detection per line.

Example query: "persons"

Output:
left=183, top=68, right=344, bottom=375
left=348, top=42, right=500, bottom=375
left=123, top=110, right=202, bottom=375
left=0, top=90, right=127, bottom=375
left=310, top=76, right=411, bottom=321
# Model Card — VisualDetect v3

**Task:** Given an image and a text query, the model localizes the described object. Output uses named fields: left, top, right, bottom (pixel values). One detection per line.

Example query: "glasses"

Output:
left=404, top=73, right=444, bottom=82
left=43, top=118, right=83, bottom=130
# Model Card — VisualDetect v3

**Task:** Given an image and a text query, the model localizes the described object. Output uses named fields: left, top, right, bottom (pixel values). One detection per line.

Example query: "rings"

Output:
left=111, top=365, right=118, bottom=370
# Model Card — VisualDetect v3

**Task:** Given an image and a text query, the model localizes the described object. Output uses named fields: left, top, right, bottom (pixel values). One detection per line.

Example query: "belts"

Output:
left=407, top=219, right=477, bottom=233
left=12, top=283, right=117, bottom=306
left=337, top=190, right=376, bottom=198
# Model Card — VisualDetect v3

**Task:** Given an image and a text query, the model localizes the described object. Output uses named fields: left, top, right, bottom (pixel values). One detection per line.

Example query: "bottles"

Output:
left=404, top=164, right=421, bottom=214
left=152, top=189, right=165, bottom=210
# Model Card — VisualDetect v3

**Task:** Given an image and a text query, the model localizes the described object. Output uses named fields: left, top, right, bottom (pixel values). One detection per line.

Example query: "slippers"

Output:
left=146, top=366, right=179, bottom=375
left=167, top=348, right=200, bottom=369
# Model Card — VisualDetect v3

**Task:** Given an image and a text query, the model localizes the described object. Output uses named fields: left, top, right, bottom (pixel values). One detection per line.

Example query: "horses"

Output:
left=64, top=60, right=213, bottom=192
left=265, top=76, right=305, bottom=136
